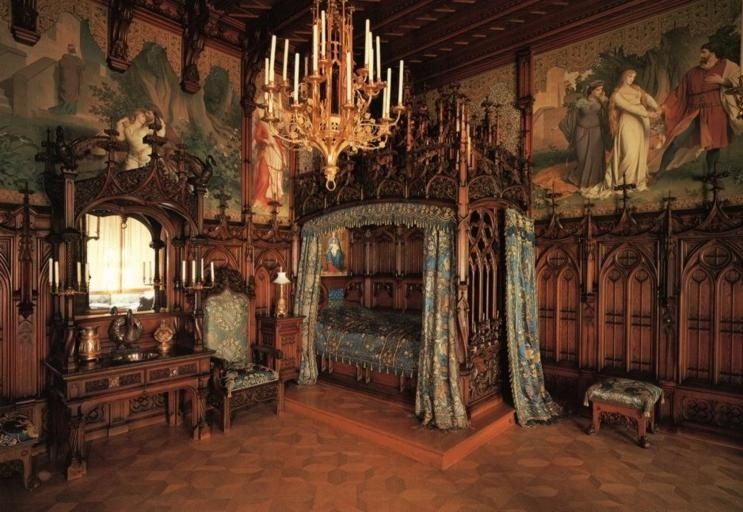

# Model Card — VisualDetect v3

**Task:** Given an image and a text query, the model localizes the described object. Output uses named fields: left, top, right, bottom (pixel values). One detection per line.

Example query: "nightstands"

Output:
left=257, top=315, right=307, bottom=381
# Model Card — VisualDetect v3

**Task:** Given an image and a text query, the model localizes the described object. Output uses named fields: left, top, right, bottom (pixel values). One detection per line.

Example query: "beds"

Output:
left=314, top=302, right=510, bottom=418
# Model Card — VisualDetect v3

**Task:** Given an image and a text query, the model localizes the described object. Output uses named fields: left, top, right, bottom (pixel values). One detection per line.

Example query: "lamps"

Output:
left=273, top=266, right=291, bottom=319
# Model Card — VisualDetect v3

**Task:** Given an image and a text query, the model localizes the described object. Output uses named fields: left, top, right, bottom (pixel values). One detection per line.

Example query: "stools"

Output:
left=582, top=377, right=665, bottom=448
left=0, top=411, right=39, bottom=491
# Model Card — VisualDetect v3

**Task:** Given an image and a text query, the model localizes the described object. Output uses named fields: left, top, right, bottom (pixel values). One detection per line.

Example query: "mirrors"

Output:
left=83, top=212, right=155, bottom=312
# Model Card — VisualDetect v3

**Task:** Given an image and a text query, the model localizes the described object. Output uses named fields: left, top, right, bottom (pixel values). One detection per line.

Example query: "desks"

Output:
left=43, top=341, right=216, bottom=481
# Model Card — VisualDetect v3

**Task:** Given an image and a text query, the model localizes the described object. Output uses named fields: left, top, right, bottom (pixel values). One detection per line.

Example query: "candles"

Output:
left=210, top=261, right=215, bottom=285
left=76, top=260, right=82, bottom=292
left=191, top=259, right=196, bottom=288
left=48, top=258, right=53, bottom=292
left=84, top=263, right=89, bottom=290
left=182, top=258, right=186, bottom=290
left=200, top=257, right=204, bottom=286
left=54, top=259, right=60, bottom=293
left=258, top=0, right=405, bottom=191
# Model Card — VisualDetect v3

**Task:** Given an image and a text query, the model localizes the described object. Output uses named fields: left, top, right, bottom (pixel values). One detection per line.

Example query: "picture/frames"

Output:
left=0, top=0, right=296, bottom=229
left=518, top=0, right=743, bottom=222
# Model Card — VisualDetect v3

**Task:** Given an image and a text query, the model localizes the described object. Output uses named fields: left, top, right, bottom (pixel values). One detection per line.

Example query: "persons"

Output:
left=325, top=232, right=346, bottom=272
left=577, top=69, right=663, bottom=200
left=560, top=80, right=613, bottom=188
left=649, top=41, right=743, bottom=185
left=253, top=121, right=287, bottom=212
left=145, top=104, right=180, bottom=172
left=116, top=108, right=165, bottom=172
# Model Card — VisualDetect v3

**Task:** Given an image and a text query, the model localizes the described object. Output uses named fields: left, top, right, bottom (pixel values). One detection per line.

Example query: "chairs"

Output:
left=202, top=266, right=284, bottom=432
